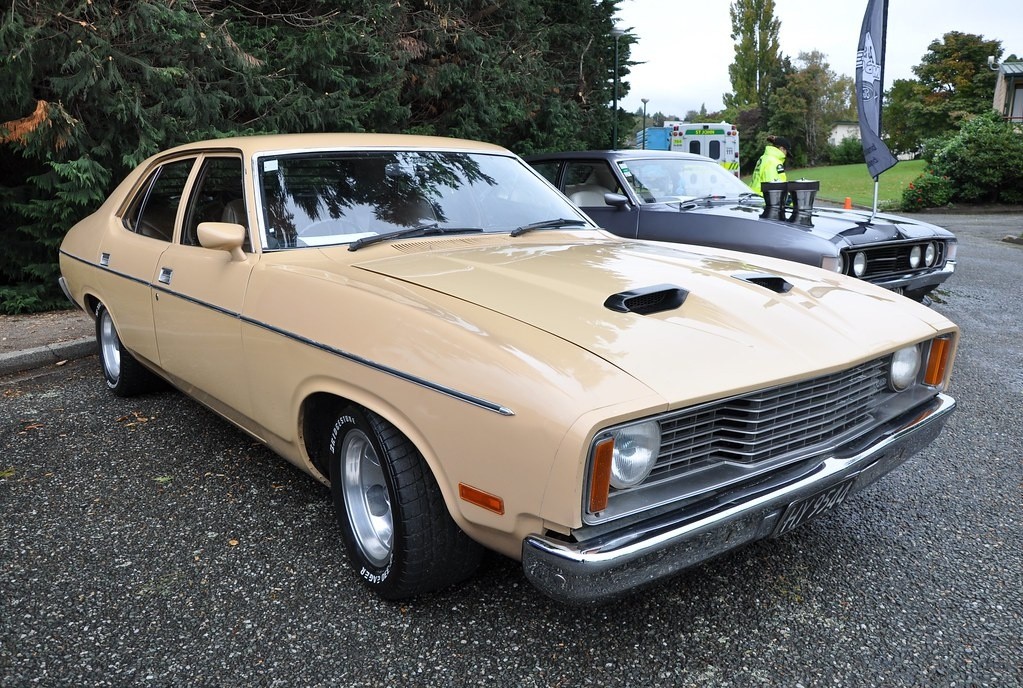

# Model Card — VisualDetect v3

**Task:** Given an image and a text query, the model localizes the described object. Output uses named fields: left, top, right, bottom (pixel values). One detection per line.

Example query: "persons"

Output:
left=750, top=136, right=793, bottom=206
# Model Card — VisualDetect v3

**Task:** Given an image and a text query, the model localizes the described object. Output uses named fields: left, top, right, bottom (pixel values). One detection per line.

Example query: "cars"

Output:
left=58, top=133, right=961, bottom=614
left=522, top=147, right=961, bottom=298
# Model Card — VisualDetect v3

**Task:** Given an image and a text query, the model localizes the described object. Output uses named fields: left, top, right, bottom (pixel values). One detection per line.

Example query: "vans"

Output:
left=669, top=120, right=743, bottom=186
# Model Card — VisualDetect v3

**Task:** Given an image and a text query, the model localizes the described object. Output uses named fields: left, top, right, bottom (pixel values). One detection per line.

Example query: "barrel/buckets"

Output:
left=789, top=190, right=816, bottom=210
left=763, top=190, right=785, bottom=205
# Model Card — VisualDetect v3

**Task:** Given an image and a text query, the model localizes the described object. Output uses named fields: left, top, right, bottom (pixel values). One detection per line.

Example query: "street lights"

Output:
left=608, top=28, right=625, bottom=151
left=639, top=95, right=651, bottom=152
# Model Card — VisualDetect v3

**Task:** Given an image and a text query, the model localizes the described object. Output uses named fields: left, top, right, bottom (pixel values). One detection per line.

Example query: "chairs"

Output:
left=222, top=198, right=251, bottom=253
left=569, top=191, right=606, bottom=205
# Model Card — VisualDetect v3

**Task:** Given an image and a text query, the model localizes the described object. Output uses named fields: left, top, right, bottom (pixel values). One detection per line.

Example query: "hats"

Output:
left=773, top=136, right=793, bottom=158
